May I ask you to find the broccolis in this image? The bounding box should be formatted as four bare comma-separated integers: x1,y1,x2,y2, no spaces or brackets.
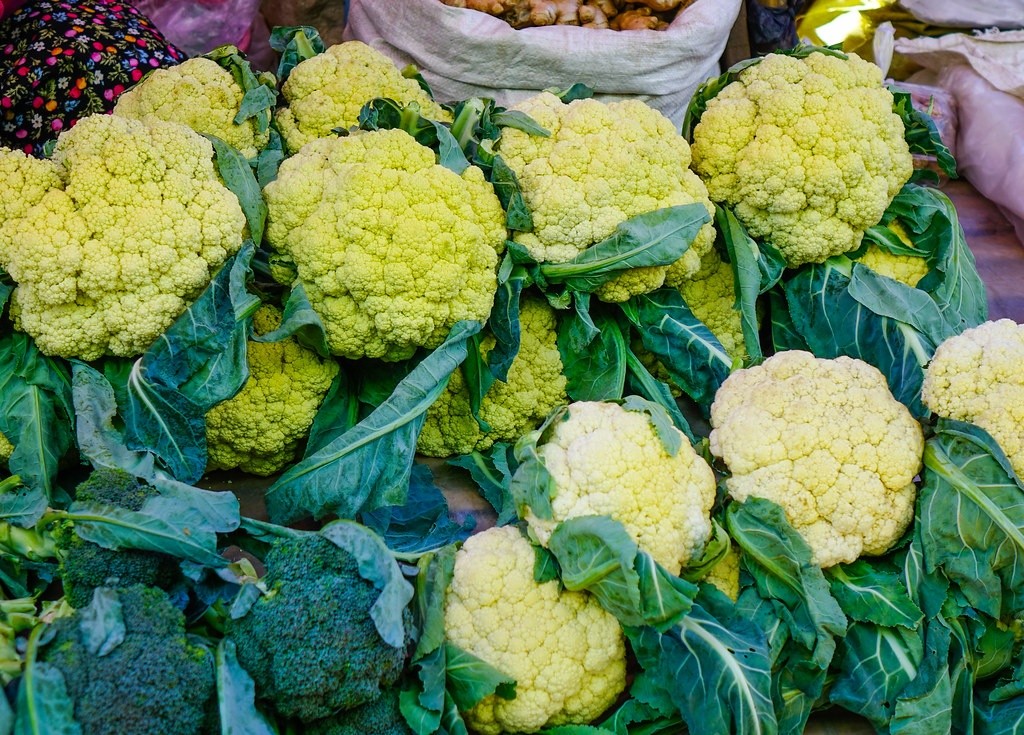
0,41,1024,735
